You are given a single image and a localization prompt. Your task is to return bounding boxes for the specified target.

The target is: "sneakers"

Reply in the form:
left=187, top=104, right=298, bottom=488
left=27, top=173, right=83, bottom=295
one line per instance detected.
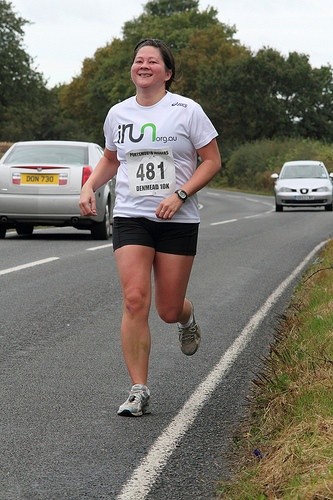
left=176, top=297, right=202, bottom=357
left=117, top=384, right=154, bottom=416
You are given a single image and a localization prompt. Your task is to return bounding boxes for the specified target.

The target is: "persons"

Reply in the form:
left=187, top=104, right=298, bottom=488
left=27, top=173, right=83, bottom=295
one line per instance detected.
left=77, top=38, right=223, bottom=417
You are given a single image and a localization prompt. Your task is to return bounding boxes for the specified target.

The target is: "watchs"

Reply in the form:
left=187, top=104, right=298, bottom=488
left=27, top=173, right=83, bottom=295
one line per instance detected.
left=175, top=189, right=187, bottom=203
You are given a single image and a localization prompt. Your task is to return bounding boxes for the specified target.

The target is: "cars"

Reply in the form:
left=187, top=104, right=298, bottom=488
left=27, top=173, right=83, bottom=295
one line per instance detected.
left=0, top=141, right=116, bottom=241
left=270, top=160, right=333, bottom=212
